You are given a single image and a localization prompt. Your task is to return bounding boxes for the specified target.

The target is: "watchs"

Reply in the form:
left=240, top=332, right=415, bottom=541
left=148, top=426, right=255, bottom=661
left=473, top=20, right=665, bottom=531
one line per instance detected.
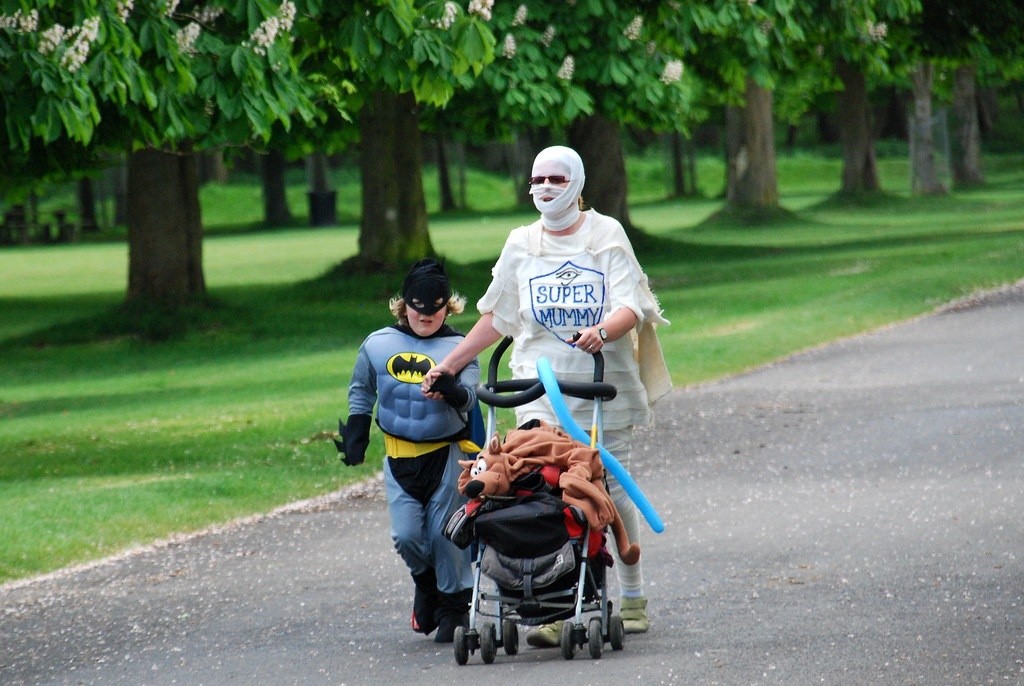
left=597, top=324, right=607, bottom=343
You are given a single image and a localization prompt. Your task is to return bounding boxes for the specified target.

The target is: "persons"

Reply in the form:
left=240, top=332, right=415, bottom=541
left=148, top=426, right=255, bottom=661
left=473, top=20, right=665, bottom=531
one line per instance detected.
left=335, top=263, right=487, bottom=642
left=424, top=146, right=673, bottom=647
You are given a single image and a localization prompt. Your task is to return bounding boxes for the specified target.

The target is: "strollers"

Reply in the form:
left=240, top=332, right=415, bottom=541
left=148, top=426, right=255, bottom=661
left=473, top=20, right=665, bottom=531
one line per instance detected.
left=453, top=332, right=625, bottom=665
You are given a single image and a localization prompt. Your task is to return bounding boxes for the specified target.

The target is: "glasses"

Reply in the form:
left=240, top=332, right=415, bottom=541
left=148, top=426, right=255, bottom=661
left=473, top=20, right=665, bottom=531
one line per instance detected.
left=530, top=175, right=569, bottom=186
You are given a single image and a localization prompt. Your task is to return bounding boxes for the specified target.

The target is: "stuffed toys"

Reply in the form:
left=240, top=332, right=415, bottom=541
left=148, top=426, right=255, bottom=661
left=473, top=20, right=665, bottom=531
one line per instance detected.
left=457, top=426, right=641, bottom=567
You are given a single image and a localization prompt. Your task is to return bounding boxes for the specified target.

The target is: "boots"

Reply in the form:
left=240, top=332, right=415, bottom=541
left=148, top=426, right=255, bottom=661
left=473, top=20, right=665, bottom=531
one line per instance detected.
left=410, top=566, right=474, bottom=643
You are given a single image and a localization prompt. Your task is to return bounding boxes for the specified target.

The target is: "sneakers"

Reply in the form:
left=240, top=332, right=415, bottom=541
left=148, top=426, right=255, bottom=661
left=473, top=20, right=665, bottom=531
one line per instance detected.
left=619, top=595, right=651, bottom=634
left=527, top=620, right=571, bottom=647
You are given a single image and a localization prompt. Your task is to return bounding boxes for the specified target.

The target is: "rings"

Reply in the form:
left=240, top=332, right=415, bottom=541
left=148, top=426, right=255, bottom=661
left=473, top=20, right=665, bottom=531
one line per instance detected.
left=589, top=345, right=594, bottom=351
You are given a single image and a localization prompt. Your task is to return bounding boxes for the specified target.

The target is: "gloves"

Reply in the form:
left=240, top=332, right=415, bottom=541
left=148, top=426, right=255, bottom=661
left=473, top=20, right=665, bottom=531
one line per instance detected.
left=428, top=373, right=469, bottom=408
left=335, top=413, right=370, bottom=467
